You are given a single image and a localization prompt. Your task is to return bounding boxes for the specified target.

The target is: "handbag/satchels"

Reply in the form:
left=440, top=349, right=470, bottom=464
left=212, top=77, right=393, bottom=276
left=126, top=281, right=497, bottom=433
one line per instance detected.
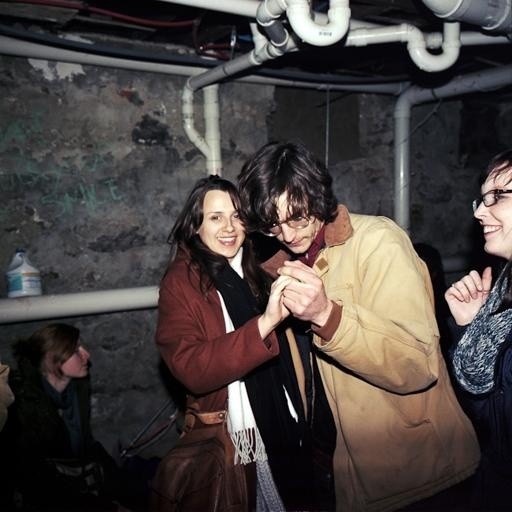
left=114, top=394, right=183, bottom=498
left=150, top=388, right=251, bottom=512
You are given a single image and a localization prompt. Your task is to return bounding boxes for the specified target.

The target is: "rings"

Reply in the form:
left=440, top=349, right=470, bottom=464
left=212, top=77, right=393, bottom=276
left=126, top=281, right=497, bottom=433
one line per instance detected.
left=452, top=281, right=456, bottom=287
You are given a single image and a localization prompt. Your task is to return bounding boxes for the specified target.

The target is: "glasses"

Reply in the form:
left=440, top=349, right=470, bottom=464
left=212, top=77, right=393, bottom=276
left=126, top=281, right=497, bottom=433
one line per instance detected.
left=257, top=206, right=310, bottom=237
left=472, top=188, right=512, bottom=208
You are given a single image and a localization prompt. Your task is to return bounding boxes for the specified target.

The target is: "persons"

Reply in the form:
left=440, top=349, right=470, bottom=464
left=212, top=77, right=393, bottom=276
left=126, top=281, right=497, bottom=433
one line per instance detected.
left=1, top=324, right=130, bottom=512
left=156, top=175, right=302, bottom=512
left=237, top=141, right=487, bottom=512
left=444, top=149, right=512, bottom=512
